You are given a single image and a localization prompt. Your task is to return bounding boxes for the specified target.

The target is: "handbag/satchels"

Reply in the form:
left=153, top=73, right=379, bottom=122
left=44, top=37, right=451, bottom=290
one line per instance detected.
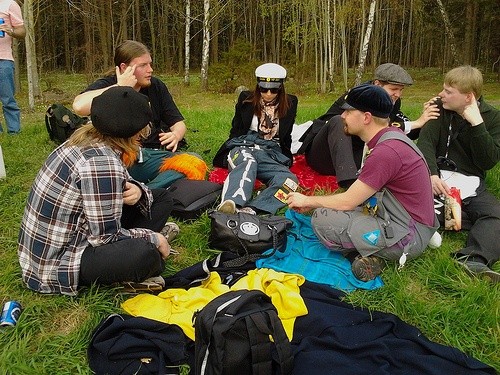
left=162, top=177, right=224, bottom=224
left=207, top=209, right=293, bottom=255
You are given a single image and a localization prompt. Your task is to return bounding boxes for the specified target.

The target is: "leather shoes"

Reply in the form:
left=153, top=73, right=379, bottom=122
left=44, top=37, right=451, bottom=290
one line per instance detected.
left=454, top=259, right=500, bottom=284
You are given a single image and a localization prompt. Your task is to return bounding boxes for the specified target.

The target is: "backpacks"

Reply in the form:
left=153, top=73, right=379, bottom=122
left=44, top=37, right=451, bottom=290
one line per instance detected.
left=192, top=289, right=295, bottom=375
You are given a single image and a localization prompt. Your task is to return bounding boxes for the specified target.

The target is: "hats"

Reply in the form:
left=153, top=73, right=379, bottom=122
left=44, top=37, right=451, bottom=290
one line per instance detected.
left=373, top=63, right=414, bottom=86
left=90, top=85, right=151, bottom=138
left=341, top=84, right=393, bottom=118
left=254, top=63, right=287, bottom=88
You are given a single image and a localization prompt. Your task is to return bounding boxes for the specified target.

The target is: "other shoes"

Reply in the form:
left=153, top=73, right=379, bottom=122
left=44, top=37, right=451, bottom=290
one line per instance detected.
left=351, top=254, right=386, bottom=281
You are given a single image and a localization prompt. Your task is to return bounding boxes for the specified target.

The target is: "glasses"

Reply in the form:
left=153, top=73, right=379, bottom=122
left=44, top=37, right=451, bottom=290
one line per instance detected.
left=259, top=85, right=281, bottom=94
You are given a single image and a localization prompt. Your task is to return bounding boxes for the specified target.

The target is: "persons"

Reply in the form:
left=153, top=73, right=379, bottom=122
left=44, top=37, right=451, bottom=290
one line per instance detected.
left=16, top=84, right=171, bottom=298
left=211, top=62, right=300, bottom=217
left=72, top=40, right=204, bottom=193
left=416, top=63, right=500, bottom=286
left=297, top=61, right=443, bottom=193
left=285, top=83, right=444, bottom=282
left=0, top=1, right=26, bottom=137
